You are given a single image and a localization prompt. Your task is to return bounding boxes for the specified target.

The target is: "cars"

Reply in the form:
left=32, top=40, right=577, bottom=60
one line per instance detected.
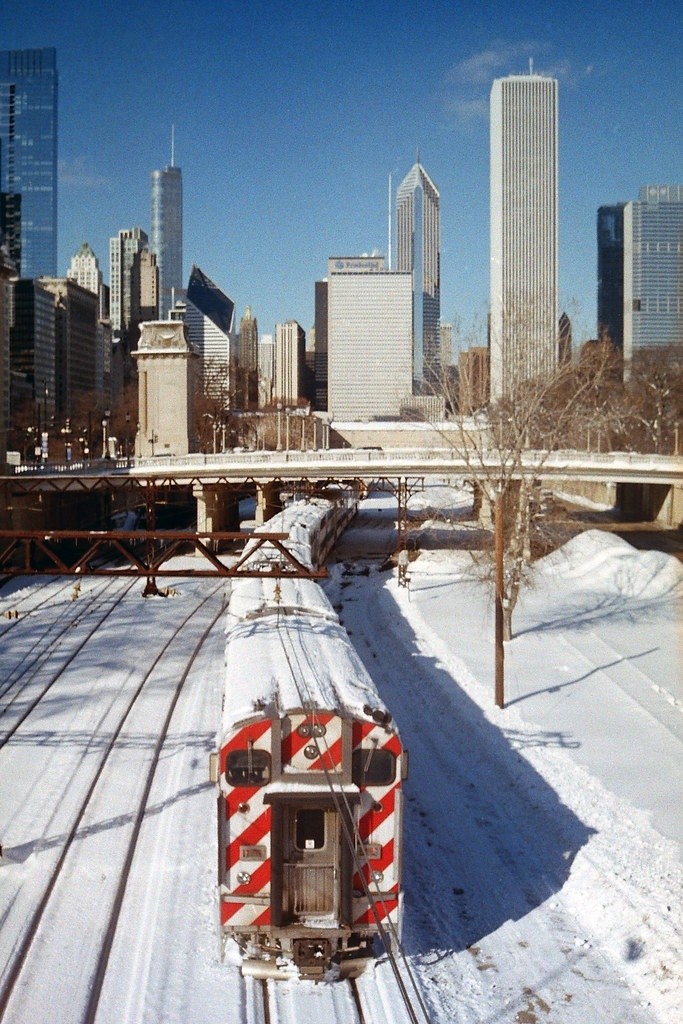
left=354, top=447, right=383, bottom=453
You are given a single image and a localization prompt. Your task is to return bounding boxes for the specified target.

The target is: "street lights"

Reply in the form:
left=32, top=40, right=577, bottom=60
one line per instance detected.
left=313, top=417, right=318, bottom=452
left=322, top=424, right=330, bottom=449
left=221, top=424, right=226, bottom=453
left=285, top=407, right=291, bottom=461
left=301, top=416, right=306, bottom=452
left=203, top=413, right=217, bottom=453
left=276, top=402, right=284, bottom=451
left=101, top=420, right=109, bottom=459
left=124, top=411, right=132, bottom=467
left=212, top=424, right=217, bottom=454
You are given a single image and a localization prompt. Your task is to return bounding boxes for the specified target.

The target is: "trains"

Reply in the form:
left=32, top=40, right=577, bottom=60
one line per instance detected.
left=211, top=477, right=411, bottom=982
